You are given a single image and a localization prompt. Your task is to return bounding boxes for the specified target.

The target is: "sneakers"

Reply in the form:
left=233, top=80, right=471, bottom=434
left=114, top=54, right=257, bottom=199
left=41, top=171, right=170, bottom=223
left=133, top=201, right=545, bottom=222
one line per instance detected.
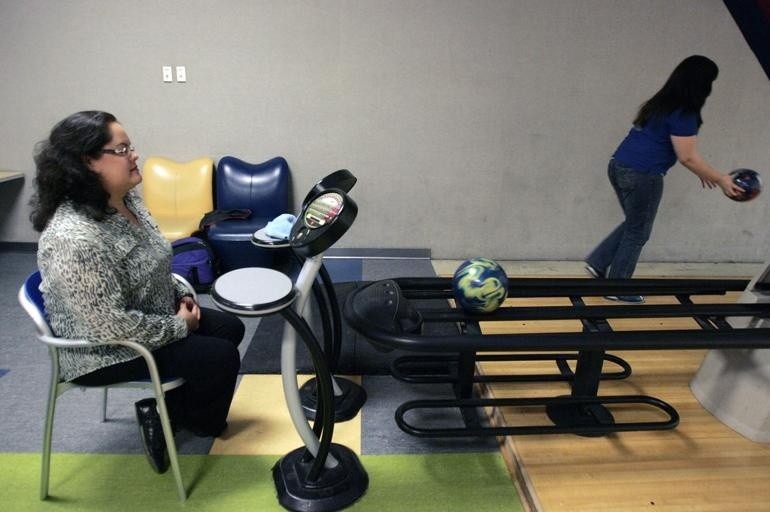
left=163, top=401, right=226, bottom=438
left=583, top=263, right=605, bottom=279
left=605, top=295, right=644, bottom=303
left=135, top=398, right=171, bottom=475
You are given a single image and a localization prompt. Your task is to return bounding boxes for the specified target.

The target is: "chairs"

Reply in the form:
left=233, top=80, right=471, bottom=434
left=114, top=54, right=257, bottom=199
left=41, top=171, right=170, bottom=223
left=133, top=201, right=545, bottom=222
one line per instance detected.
left=17, top=270, right=200, bottom=504
left=141, top=155, right=299, bottom=293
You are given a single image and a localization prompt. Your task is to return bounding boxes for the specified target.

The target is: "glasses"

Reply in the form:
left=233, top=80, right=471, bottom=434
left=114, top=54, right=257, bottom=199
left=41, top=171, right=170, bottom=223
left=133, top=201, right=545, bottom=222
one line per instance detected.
left=97, top=143, right=136, bottom=158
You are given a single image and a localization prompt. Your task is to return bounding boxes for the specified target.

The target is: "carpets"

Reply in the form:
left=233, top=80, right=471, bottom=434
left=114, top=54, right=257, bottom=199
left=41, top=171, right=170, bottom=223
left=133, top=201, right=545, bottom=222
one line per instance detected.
left=0, top=451, right=528, bottom=512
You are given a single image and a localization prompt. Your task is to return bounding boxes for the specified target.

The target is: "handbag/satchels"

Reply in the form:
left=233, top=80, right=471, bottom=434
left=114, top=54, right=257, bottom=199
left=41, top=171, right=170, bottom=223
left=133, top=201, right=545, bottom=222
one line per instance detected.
left=171, top=231, right=220, bottom=295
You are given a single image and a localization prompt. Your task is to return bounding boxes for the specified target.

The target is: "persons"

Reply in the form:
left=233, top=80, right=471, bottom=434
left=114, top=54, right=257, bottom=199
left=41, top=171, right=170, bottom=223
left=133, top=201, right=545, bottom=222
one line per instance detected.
left=585, top=54, right=748, bottom=303
left=29, top=110, right=246, bottom=474
